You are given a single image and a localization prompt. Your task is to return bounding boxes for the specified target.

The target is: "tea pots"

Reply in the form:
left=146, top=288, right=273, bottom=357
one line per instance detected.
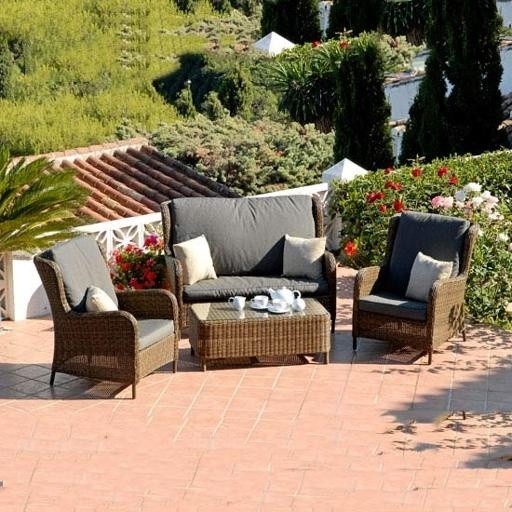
left=270, top=287, right=301, bottom=305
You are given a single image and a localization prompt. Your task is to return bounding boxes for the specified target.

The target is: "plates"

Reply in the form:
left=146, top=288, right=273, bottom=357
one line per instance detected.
left=268, top=308, right=290, bottom=313
left=249, top=304, right=272, bottom=309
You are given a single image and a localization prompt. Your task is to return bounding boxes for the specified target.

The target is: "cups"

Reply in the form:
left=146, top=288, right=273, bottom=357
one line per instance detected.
left=271, top=299, right=288, bottom=310
left=250, top=296, right=268, bottom=306
left=229, top=296, right=245, bottom=309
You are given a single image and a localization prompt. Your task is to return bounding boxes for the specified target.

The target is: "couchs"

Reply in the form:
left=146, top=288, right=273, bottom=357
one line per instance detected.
left=34, top=236, right=180, bottom=399
left=160, top=194, right=338, bottom=334
left=351, top=212, right=479, bottom=364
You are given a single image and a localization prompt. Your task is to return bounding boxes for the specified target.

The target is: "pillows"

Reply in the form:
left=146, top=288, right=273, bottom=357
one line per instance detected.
left=84, top=285, right=119, bottom=315
left=282, top=233, right=326, bottom=282
left=405, top=251, right=455, bottom=302
left=172, top=235, right=216, bottom=285
